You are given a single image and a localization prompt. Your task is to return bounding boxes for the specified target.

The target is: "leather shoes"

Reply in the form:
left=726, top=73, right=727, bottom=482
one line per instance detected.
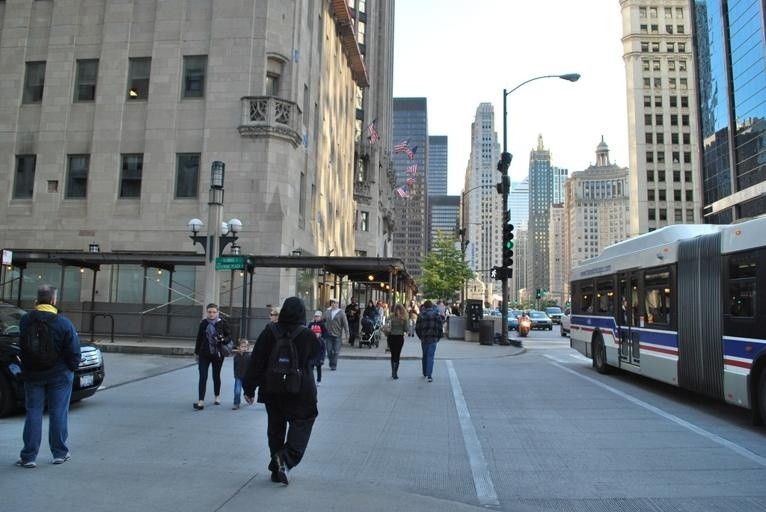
left=215, top=402, right=220, bottom=405
left=193, top=403, right=203, bottom=410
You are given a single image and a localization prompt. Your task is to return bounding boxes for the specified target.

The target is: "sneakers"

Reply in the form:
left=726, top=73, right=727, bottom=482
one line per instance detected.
left=52, top=453, right=71, bottom=464
left=16, top=459, right=36, bottom=468
left=268, top=452, right=289, bottom=484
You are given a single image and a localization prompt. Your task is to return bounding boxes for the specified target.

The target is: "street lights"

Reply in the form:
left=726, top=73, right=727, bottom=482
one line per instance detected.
left=460, top=184, right=497, bottom=312
left=88, top=242, right=100, bottom=340
left=186, top=158, right=243, bottom=344
left=0, top=247, right=12, bottom=301
left=499, top=73, right=581, bottom=340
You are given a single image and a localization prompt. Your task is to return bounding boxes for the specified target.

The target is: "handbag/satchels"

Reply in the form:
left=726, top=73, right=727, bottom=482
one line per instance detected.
left=220, top=339, right=233, bottom=358
left=384, top=320, right=392, bottom=336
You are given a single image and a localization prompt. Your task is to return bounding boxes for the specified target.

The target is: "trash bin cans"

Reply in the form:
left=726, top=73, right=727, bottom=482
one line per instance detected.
left=479, top=320, right=494, bottom=345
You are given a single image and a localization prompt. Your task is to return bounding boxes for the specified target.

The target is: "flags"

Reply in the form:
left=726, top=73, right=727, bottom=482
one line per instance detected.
left=366, top=119, right=417, bottom=200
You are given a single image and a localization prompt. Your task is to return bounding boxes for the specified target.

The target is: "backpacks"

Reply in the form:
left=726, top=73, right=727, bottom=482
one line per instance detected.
left=267, top=322, right=307, bottom=396
left=22, top=311, right=64, bottom=373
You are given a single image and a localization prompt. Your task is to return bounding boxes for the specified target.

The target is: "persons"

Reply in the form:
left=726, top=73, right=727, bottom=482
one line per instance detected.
left=245, top=298, right=323, bottom=486
left=586, top=299, right=671, bottom=328
left=518, top=312, right=532, bottom=320
left=234, top=294, right=460, bottom=410
left=14, top=285, right=80, bottom=469
left=191, top=302, right=231, bottom=410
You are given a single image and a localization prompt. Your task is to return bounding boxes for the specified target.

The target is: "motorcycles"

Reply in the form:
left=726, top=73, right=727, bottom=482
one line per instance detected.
left=516, top=316, right=532, bottom=336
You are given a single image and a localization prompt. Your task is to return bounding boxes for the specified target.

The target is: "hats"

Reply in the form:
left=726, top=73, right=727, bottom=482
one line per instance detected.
left=315, top=311, right=322, bottom=317
left=312, top=325, right=321, bottom=333
left=331, top=299, right=338, bottom=303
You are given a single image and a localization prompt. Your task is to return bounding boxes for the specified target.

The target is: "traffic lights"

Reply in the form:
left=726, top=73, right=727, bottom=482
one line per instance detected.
left=541, top=290, right=546, bottom=296
left=490, top=268, right=500, bottom=278
left=505, top=268, right=512, bottom=279
left=535, top=288, right=541, bottom=299
left=505, top=224, right=514, bottom=266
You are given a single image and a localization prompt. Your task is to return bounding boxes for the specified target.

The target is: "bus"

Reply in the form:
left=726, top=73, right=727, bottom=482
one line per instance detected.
left=569, top=213, right=766, bottom=421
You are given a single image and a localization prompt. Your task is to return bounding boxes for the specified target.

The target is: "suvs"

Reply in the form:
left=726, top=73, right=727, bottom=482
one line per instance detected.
left=560, top=308, right=571, bottom=337
left=0, top=299, right=104, bottom=415
left=545, top=307, right=562, bottom=325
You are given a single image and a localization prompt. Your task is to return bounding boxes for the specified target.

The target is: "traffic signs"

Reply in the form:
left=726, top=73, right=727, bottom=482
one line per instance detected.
left=216, top=257, right=243, bottom=263
left=216, top=264, right=243, bottom=270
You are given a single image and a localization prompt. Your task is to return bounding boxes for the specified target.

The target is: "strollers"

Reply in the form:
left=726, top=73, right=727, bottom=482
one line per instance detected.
left=357, top=318, right=381, bottom=349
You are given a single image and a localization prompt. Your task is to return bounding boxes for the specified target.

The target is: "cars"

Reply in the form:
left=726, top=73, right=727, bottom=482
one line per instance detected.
left=482, top=307, right=553, bottom=331
